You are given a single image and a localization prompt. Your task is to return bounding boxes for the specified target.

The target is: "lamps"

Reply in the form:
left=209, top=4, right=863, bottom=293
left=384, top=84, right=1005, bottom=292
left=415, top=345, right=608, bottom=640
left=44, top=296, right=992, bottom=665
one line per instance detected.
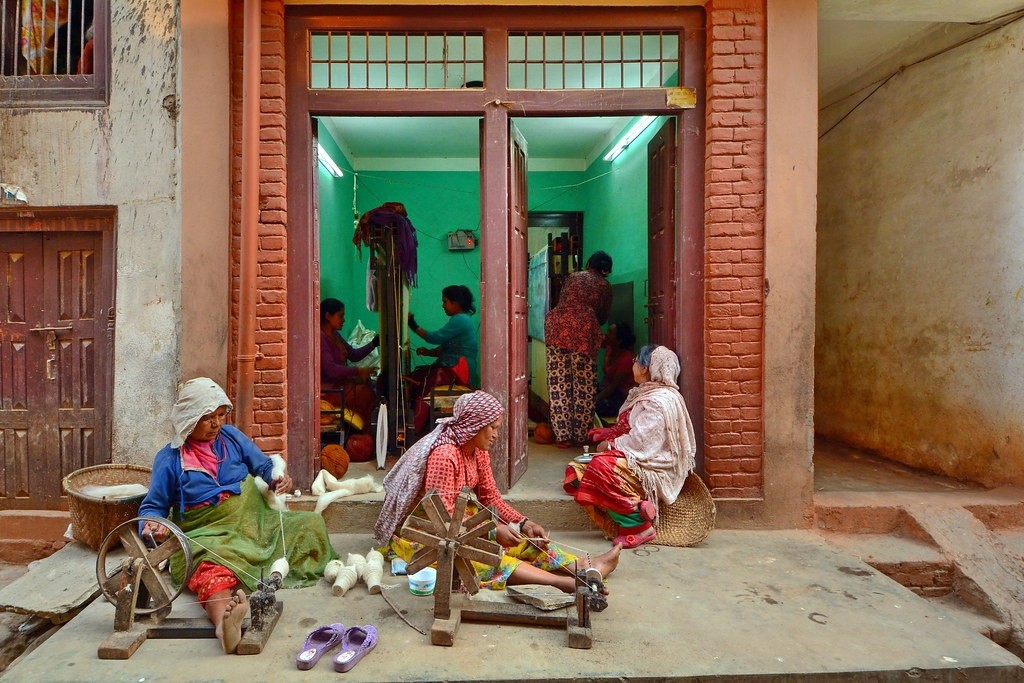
left=318, top=141, right=344, bottom=178
left=603, top=116, right=658, bottom=162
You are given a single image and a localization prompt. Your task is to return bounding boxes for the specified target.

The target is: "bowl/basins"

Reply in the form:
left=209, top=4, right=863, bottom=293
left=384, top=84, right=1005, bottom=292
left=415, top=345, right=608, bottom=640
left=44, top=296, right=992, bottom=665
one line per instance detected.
left=407, top=567, right=438, bottom=596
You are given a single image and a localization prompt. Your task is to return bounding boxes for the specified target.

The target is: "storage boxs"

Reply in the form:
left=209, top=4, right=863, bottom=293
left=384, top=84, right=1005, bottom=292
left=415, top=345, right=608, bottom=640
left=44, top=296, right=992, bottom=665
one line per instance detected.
left=553, top=237, right=563, bottom=252
left=551, top=254, right=561, bottom=273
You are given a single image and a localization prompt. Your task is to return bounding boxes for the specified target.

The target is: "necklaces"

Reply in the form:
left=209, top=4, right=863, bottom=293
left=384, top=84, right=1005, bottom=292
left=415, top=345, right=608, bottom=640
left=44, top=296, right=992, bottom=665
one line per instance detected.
left=184, top=431, right=227, bottom=464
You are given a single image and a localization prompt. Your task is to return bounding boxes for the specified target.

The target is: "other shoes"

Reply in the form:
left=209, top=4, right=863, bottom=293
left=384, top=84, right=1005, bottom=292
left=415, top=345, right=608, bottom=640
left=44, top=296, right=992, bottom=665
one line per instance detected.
left=556, top=439, right=582, bottom=448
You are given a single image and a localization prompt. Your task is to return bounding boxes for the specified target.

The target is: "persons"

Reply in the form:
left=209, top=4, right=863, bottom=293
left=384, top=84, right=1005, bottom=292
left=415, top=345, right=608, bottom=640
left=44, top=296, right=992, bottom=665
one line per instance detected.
left=407, top=285, right=479, bottom=438
left=593, top=320, right=638, bottom=418
left=563, top=343, right=697, bottom=548
left=137, top=377, right=325, bottom=655
left=320, top=297, right=380, bottom=435
left=372, top=389, right=623, bottom=594
left=543, top=250, right=614, bottom=449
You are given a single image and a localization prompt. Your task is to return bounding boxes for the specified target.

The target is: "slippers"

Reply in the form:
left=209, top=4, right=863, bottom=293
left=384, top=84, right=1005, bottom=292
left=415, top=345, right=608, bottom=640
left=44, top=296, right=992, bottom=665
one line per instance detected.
left=297, top=623, right=345, bottom=670
left=639, top=500, right=656, bottom=521
left=613, top=531, right=656, bottom=547
left=333, top=625, right=378, bottom=672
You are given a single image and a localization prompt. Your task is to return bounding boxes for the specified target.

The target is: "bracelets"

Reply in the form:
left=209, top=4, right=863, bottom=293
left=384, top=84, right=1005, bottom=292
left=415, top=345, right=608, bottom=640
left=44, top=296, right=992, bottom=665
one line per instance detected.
left=607, top=441, right=612, bottom=451
left=519, top=518, right=528, bottom=533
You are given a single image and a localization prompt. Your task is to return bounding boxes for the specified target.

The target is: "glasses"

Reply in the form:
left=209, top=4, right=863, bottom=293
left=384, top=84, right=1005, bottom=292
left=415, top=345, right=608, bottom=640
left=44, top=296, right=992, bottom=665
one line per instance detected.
left=633, top=353, right=643, bottom=363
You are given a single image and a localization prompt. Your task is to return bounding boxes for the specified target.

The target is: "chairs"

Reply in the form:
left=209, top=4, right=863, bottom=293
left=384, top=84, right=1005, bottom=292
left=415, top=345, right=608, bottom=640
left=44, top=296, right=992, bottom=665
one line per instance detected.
left=320, top=388, right=348, bottom=451
left=422, top=368, right=477, bottom=434
